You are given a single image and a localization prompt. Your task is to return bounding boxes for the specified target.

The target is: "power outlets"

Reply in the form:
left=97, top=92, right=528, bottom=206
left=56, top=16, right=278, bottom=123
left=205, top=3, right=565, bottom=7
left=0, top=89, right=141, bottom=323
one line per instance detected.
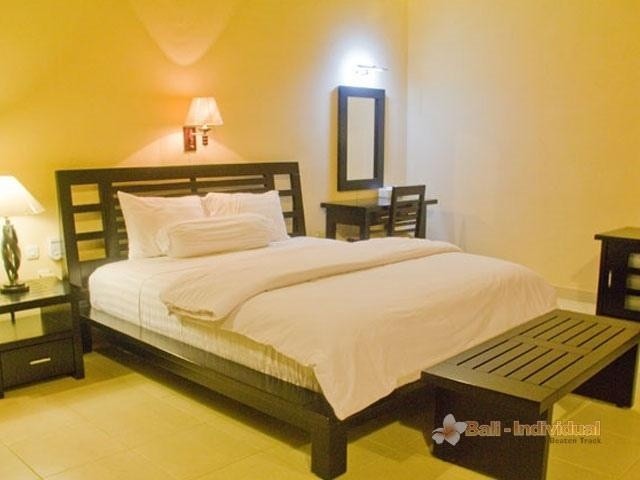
left=25, top=243, right=39, bottom=260
left=48, top=240, right=66, bottom=260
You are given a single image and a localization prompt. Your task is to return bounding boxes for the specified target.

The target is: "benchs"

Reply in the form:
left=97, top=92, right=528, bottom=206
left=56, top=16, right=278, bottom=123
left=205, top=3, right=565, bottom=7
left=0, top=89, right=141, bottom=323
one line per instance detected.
left=421, top=309, right=640, bottom=480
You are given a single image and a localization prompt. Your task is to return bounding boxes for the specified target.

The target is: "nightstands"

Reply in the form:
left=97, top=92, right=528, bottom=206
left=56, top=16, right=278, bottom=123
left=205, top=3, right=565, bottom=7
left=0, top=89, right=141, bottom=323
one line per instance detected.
left=0, top=276, right=85, bottom=399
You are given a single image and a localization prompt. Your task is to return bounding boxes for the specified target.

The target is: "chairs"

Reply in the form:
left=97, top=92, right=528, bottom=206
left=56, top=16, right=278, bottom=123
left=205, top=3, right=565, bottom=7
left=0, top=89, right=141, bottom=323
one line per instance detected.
left=387, top=184, right=426, bottom=239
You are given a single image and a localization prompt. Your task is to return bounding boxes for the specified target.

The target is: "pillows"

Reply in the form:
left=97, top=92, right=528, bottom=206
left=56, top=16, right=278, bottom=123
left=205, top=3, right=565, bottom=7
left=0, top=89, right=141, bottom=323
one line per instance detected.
left=117, top=190, right=205, bottom=260
left=203, top=189, right=291, bottom=244
left=156, top=213, right=276, bottom=260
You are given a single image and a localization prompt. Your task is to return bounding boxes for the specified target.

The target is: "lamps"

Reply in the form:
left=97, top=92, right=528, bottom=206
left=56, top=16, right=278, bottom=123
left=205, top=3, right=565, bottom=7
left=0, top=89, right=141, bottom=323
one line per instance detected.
left=182, top=96, right=223, bottom=152
left=0, top=175, right=46, bottom=295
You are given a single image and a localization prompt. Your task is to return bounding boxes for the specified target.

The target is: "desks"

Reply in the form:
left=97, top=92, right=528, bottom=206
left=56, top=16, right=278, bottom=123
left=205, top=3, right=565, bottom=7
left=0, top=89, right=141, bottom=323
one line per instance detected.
left=320, top=196, right=438, bottom=242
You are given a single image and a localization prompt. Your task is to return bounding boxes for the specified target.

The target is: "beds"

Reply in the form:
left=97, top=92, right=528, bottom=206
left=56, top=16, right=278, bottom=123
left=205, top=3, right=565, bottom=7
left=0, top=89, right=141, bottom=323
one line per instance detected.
left=56, top=161, right=558, bottom=480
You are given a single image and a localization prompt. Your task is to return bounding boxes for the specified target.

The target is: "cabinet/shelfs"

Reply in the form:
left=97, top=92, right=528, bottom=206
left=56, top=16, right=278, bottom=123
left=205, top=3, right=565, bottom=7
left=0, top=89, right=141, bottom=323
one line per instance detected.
left=587, top=227, right=640, bottom=410
